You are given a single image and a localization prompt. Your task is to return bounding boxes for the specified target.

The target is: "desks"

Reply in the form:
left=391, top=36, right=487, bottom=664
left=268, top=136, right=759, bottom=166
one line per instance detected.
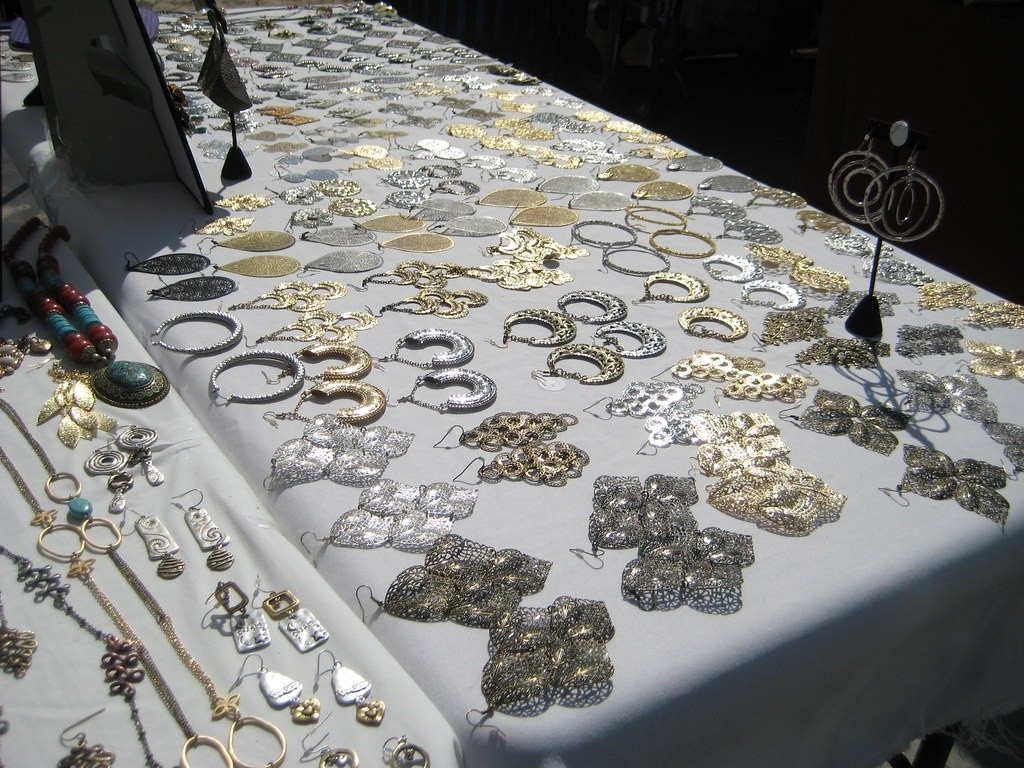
left=0, top=0, right=1024, bottom=768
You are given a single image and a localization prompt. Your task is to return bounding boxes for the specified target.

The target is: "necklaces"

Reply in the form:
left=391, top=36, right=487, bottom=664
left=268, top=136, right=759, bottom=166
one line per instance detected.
left=1, top=218, right=170, bottom=410
left=0, top=396, right=288, bottom=768
